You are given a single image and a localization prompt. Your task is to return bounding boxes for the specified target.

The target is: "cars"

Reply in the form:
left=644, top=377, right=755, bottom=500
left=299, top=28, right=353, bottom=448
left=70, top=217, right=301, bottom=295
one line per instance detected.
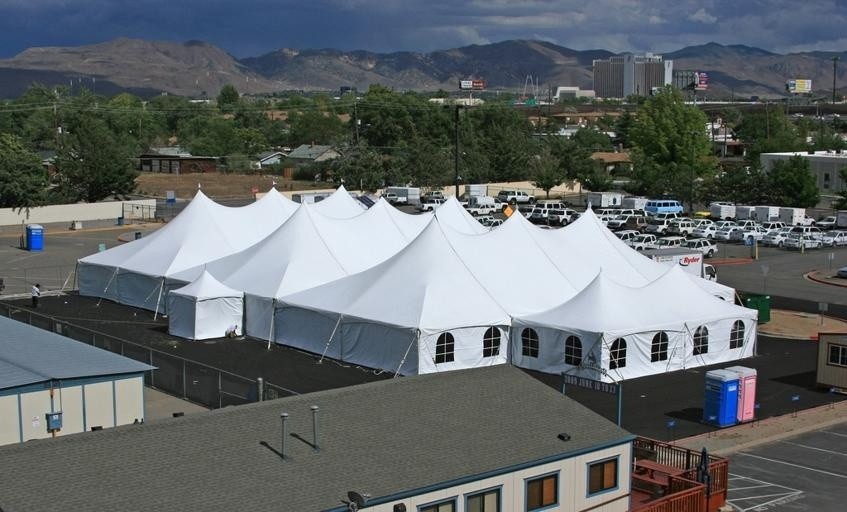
left=593, top=199, right=847, bottom=258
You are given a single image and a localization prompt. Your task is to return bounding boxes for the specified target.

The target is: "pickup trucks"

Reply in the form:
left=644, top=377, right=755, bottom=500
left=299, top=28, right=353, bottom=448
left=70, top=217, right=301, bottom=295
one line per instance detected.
left=380, top=192, right=409, bottom=206
left=414, top=199, right=447, bottom=213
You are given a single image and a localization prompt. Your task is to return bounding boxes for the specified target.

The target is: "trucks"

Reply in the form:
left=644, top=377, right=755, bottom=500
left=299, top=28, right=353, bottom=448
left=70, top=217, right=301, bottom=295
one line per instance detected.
left=636, top=247, right=718, bottom=283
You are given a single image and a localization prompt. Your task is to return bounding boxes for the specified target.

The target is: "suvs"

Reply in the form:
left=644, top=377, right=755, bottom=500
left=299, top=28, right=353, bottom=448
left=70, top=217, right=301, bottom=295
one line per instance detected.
left=421, top=191, right=444, bottom=202
left=462, top=189, right=585, bottom=231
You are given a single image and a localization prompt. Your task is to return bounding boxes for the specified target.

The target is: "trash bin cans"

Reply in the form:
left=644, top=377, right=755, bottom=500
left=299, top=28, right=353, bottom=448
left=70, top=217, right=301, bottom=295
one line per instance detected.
left=135, top=233, right=142, bottom=239
left=25, top=223, right=44, bottom=250
left=118, top=217, right=123, bottom=226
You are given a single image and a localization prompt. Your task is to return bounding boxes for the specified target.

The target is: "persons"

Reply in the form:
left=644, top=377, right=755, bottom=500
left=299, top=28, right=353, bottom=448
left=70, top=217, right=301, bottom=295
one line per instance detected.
left=32, top=283, right=40, bottom=307
